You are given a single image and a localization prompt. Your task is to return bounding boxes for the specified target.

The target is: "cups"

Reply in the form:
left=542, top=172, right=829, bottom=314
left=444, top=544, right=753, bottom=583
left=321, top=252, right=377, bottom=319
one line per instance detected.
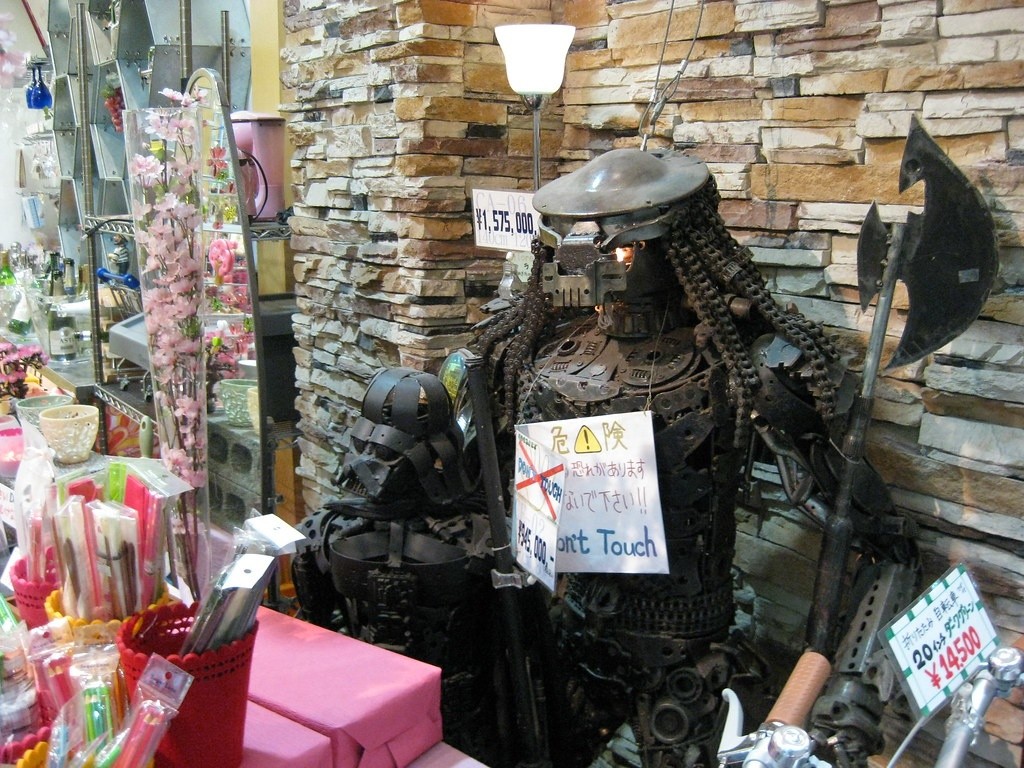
left=45, top=580, right=169, bottom=644
left=10, top=547, right=60, bottom=629
left=117, top=601, right=259, bottom=768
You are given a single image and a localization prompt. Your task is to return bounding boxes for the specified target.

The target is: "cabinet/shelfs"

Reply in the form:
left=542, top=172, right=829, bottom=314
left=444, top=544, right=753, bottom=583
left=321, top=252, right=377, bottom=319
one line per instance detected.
left=84, top=215, right=296, bottom=456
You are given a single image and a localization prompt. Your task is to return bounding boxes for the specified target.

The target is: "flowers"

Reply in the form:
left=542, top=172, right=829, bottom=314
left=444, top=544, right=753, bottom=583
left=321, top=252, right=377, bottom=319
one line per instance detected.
left=0, top=342, right=49, bottom=399
left=122, top=88, right=243, bottom=601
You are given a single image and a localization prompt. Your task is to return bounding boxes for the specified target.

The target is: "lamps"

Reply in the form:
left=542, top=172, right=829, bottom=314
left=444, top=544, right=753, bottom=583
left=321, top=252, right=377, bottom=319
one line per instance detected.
left=494, top=23, right=576, bottom=195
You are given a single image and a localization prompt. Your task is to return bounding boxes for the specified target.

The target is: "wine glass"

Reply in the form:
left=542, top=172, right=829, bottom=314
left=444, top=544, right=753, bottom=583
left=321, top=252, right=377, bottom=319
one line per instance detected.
left=26, top=62, right=52, bottom=109
left=30, top=137, right=59, bottom=180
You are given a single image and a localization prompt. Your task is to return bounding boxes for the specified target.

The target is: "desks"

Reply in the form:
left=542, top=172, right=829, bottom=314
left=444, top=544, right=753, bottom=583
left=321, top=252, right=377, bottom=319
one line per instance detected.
left=0, top=329, right=96, bottom=405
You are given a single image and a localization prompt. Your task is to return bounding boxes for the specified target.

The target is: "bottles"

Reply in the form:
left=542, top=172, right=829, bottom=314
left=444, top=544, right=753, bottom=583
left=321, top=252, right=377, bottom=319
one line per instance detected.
left=0, top=242, right=83, bottom=362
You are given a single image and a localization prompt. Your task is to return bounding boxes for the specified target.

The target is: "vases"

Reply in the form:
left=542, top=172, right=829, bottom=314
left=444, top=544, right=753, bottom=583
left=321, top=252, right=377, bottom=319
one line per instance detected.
left=205, top=381, right=216, bottom=414
